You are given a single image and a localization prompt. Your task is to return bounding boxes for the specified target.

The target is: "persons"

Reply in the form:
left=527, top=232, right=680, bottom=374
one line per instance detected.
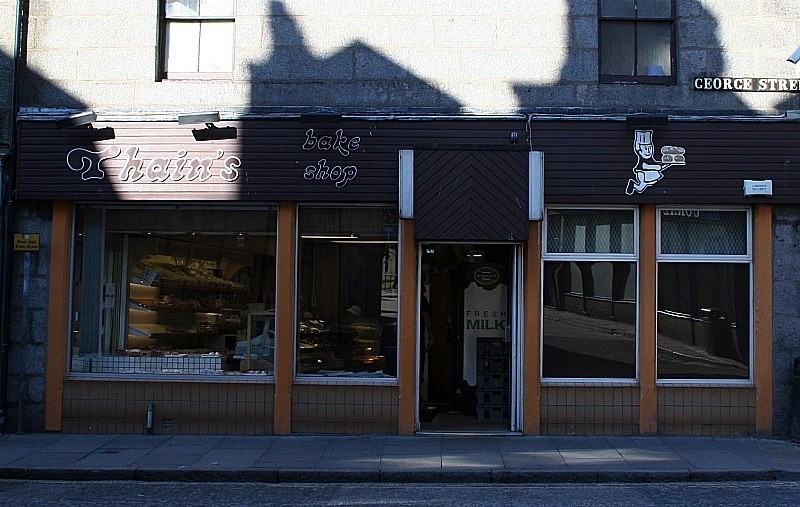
left=420, top=284, right=435, bottom=403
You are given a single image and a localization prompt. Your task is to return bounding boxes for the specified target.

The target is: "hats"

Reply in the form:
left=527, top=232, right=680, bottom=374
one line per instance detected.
left=347, top=305, right=362, bottom=316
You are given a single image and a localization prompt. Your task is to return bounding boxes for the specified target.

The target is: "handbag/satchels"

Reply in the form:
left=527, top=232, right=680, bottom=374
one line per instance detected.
left=423, top=319, right=436, bottom=351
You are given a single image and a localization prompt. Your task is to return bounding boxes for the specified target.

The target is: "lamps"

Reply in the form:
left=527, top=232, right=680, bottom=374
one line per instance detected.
left=54, top=111, right=101, bottom=134
left=179, top=112, right=220, bottom=130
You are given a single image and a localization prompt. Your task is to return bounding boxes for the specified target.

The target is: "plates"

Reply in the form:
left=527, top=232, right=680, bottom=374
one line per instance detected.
left=200, top=354, right=222, bottom=357
left=164, top=354, right=187, bottom=357
